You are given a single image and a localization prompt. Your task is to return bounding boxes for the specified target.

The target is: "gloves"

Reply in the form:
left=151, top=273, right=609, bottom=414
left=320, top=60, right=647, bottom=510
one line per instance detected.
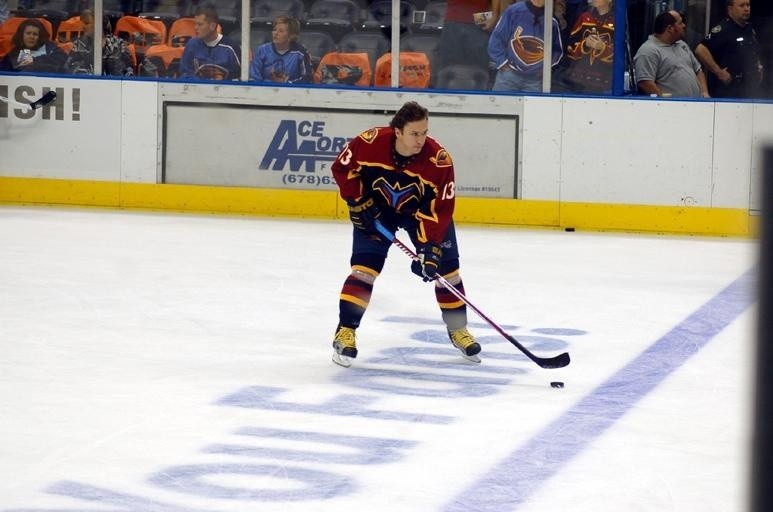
left=347, top=194, right=383, bottom=235
left=411, top=241, right=444, bottom=282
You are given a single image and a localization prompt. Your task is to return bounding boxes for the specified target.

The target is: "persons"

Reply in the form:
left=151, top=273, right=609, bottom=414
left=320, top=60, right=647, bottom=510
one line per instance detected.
left=62, top=7, right=134, bottom=78
left=178, top=5, right=242, bottom=82
left=329, top=100, right=485, bottom=357
left=486, top=0, right=563, bottom=93
left=0, top=19, right=67, bottom=74
left=693, top=0, right=762, bottom=99
left=248, top=16, right=312, bottom=83
left=633, top=11, right=711, bottom=99
left=436, top=1, right=494, bottom=69
left=565, top=0, right=615, bottom=94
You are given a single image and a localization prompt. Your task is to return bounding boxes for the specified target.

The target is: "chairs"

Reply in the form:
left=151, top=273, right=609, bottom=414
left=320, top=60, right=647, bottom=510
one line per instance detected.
left=0, top=0, right=497, bottom=93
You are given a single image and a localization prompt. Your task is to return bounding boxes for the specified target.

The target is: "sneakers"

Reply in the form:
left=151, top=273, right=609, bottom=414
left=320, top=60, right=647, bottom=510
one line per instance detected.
left=333, top=324, right=358, bottom=359
left=447, top=326, right=481, bottom=357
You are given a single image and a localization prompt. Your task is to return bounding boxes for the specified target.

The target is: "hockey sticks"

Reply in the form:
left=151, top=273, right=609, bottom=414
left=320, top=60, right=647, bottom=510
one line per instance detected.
left=375, top=220, right=570, bottom=368
